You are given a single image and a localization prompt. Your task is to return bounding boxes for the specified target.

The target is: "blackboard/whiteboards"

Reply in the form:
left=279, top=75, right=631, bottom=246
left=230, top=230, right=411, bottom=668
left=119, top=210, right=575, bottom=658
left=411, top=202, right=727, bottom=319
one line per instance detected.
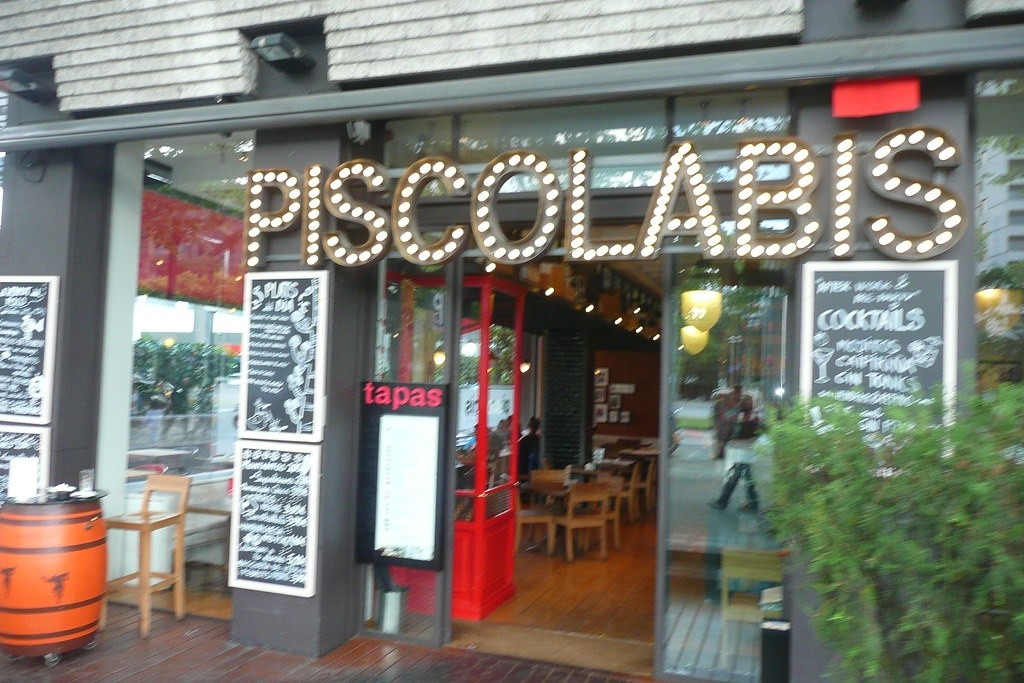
left=795, top=260, right=962, bottom=491
left=234, top=271, right=327, bottom=442
left=225, top=442, right=326, bottom=598
left=0, top=275, right=63, bottom=507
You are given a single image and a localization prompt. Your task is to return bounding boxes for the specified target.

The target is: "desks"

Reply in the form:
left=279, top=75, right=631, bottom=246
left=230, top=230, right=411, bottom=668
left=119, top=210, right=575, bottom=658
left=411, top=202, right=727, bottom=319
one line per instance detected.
left=530, top=469, right=624, bottom=541
left=595, top=458, right=636, bottom=507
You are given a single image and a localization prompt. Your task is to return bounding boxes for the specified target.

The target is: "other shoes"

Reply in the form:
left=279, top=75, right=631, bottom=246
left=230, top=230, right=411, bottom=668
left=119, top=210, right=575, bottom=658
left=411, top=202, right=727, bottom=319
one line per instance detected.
left=709, top=502, right=727, bottom=510
left=738, top=501, right=758, bottom=513
left=713, top=454, right=724, bottom=459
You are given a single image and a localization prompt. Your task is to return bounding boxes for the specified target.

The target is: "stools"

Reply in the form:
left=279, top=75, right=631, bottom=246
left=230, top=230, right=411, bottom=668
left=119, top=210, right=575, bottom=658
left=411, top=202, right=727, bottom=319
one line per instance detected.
left=97, top=474, right=193, bottom=638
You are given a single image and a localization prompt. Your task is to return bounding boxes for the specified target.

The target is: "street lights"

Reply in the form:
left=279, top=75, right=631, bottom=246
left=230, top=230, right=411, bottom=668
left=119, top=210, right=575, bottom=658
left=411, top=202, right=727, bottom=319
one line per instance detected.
left=726, top=333, right=742, bottom=388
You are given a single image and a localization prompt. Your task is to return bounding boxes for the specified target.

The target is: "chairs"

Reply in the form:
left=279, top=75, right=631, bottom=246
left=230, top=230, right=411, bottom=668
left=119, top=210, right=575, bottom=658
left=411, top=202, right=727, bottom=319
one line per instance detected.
left=577, top=476, right=625, bottom=552
left=621, top=461, right=642, bottom=522
left=557, top=482, right=611, bottom=562
left=625, top=458, right=657, bottom=508
left=720, top=547, right=787, bottom=651
left=515, top=481, right=555, bottom=557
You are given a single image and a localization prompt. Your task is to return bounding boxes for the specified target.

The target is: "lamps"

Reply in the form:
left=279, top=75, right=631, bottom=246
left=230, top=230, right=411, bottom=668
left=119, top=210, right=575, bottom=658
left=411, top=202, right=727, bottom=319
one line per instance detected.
left=0, top=70, right=47, bottom=99
left=249, top=32, right=301, bottom=62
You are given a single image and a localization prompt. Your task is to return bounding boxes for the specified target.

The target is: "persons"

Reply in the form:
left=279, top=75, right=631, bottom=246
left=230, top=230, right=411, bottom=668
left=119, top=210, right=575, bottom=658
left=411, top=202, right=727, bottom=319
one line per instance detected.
left=455, top=414, right=513, bottom=519
left=518, top=417, right=540, bottom=504
left=710, top=385, right=759, bottom=512
left=161, top=378, right=193, bottom=442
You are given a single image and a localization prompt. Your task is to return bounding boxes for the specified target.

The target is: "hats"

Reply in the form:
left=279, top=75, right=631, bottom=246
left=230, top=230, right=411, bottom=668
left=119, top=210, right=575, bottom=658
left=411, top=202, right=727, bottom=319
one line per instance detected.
left=744, top=388, right=762, bottom=411
